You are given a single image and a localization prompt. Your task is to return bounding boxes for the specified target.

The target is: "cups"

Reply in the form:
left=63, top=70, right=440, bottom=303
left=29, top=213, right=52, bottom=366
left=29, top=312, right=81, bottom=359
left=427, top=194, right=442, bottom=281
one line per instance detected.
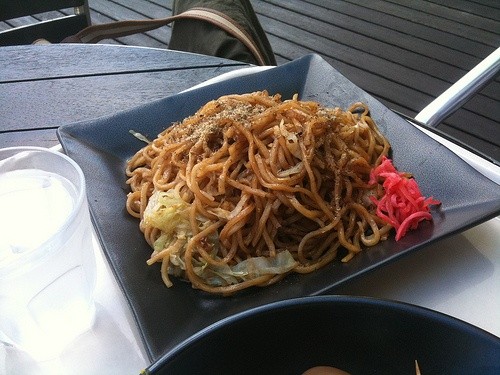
left=1, top=146, right=96, bottom=350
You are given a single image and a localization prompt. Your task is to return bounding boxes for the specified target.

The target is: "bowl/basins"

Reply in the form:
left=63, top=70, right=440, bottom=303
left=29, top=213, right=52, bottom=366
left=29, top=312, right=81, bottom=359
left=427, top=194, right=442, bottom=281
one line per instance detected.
left=143, top=295, right=499, bottom=375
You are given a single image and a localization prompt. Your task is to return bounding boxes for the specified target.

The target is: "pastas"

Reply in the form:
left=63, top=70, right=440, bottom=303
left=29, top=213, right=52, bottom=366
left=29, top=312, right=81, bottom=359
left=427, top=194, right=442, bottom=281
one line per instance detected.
left=125, top=90, right=439, bottom=293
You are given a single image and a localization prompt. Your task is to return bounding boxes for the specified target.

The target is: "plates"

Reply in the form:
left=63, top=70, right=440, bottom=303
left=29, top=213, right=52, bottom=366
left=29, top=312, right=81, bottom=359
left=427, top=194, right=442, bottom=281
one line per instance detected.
left=55, top=53, right=500, bottom=363
